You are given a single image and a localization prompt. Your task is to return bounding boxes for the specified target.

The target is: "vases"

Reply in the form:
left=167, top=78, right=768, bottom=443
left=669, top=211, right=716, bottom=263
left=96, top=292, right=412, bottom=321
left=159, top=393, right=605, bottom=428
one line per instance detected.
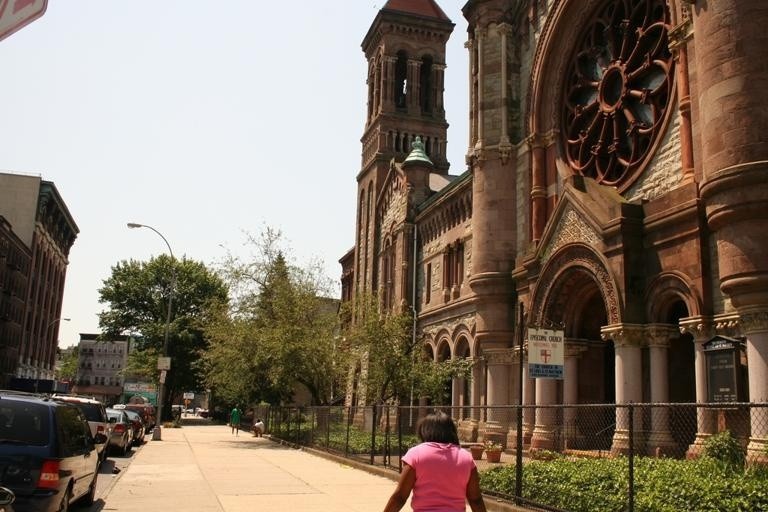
left=470, top=445, right=485, bottom=461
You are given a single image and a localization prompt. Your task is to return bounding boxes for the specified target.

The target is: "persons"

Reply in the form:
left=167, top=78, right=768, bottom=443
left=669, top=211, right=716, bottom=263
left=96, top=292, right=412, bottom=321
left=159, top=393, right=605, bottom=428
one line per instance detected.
left=230, top=403, right=242, bottom=436
left=382, top=409, right=487, bottom=511
left=252, top=418, right=265, bottom=437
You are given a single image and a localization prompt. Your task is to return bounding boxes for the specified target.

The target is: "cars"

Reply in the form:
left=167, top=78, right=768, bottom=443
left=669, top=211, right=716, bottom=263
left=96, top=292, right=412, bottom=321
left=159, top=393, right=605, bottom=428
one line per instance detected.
left=52, top=395, right=116, bottom=469
left=1, top=396, right=108, bottom=512
left=104, top=402, right=180, bottom=454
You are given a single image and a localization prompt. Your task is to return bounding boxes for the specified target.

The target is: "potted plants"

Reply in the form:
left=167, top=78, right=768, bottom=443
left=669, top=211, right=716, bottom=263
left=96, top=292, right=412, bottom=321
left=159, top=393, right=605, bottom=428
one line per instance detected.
left=485, top=440, right=502, bottom=463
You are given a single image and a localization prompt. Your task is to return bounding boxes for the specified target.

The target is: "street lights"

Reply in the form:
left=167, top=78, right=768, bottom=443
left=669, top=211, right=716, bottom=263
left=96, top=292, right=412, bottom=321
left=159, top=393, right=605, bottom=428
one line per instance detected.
left=35, top=319, right=70, bottom=393
left=128, top=224, right=173, bottom=426
